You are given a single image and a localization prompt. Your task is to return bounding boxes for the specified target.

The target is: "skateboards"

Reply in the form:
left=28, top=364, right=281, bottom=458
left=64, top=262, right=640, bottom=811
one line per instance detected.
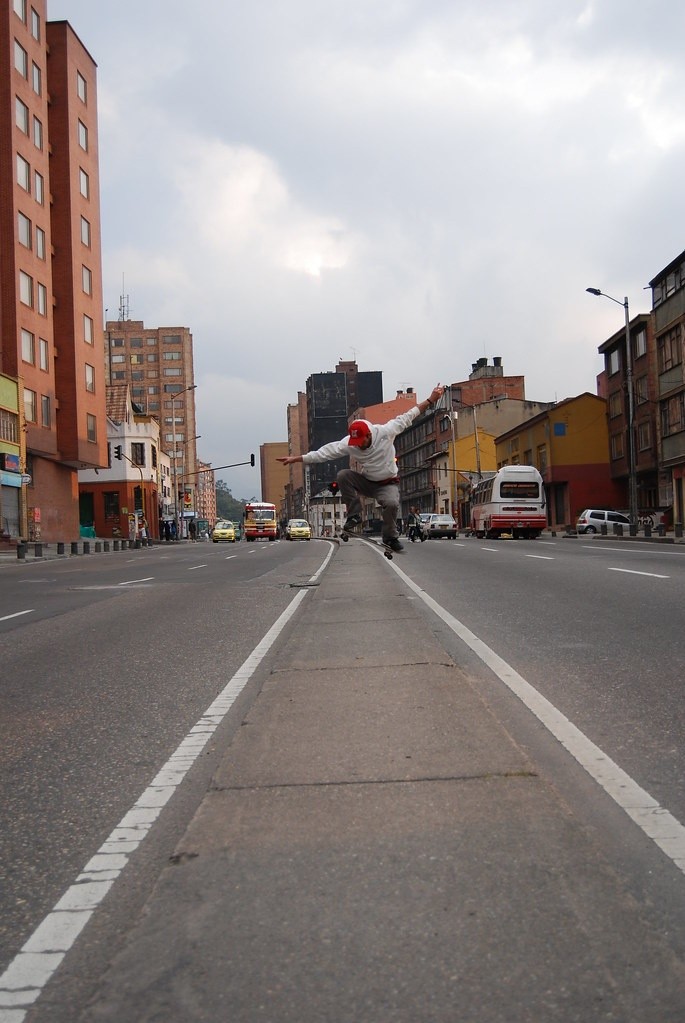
left=331, top=520, right=407, bottom=560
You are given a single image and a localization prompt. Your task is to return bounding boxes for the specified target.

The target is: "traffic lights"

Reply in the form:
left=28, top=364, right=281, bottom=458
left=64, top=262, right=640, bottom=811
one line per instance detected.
left=113, top=445, right=122, bottom=460
left=328, top=482, right=339, bottom=494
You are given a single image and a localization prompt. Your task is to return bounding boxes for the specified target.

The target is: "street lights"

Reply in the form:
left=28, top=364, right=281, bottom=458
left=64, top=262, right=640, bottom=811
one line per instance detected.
left=444, top=414, right=460, bottom=527
left=586, top=287, right=639, bottom=529
left=450, top=398, right=481, bottom=480
left=171, top=385, right=198, bottom=539
left=181, top=435, right=202, bottom=540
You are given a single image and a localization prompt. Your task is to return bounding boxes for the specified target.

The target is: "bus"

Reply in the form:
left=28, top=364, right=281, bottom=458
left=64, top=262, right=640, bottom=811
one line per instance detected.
left=468, top=465, right=548, bottom=539
left=242, top=503, right=278, bottom=542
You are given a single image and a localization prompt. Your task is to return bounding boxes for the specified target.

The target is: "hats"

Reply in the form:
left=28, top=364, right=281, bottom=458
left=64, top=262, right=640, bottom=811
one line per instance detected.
left=348, top=421, right=369, bottom=446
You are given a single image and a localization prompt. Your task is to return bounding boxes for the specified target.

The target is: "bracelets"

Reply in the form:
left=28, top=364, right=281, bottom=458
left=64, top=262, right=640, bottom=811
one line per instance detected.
left=427, top=398, right=433, bottom=406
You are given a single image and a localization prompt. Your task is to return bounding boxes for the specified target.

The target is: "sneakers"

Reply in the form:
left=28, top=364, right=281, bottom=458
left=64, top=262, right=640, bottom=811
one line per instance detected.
left=383, top=538, right=403, bottom=550
left=344, top=514, right=362, bottom=529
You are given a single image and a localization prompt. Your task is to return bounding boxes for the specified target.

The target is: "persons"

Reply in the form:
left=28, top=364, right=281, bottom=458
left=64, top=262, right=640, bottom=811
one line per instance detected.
left=138, top=524, right=146, bottom=538
left=159, top=520, right=178, bottom=541
left=276, top=382, right=446, bottom=551
left=277, top=525, right=291, bottom=540
left=406, top=506, right=426, bottom=543
left=189, top=518, right=196, bottom=540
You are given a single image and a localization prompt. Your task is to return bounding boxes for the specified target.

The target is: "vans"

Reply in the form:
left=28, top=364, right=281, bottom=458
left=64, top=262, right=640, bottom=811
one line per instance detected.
left=285, top=518, right=305, bottom=540
left=576, top=509, right=630, bottom=534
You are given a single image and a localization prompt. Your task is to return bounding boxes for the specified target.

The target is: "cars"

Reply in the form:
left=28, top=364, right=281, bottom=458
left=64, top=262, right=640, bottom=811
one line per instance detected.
left=421, top=514, right=458, bottom=539
left=416, top=513, right=437, bottom=534
left=231, top=521, right=242, bottom=540
left=212, top=521, right=236, bottom=543
left=289, top=520, right=311, bottom=541
